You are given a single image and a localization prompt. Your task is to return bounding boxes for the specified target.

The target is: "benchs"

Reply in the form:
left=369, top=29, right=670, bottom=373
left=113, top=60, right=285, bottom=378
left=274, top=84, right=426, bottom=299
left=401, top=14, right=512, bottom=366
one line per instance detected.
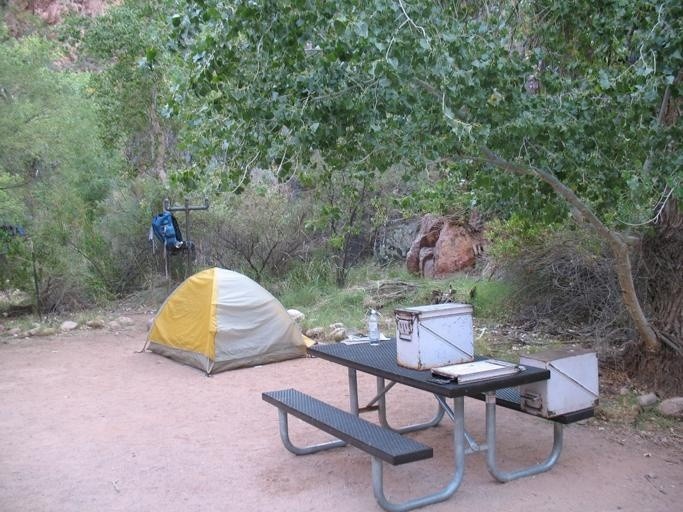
left=476, top=388, right=595, bottom=458
left=262, top=388, right=434, bottom=512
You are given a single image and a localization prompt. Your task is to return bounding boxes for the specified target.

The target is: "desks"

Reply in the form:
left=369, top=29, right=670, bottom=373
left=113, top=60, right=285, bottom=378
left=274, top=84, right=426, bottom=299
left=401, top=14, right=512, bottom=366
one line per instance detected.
left=306, top=337, right=551, bottom=476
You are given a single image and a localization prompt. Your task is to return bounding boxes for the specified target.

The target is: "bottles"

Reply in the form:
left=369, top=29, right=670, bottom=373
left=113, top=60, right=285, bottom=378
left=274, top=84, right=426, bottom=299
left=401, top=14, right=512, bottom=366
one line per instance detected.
left=368, top=310, right=380, bottom=345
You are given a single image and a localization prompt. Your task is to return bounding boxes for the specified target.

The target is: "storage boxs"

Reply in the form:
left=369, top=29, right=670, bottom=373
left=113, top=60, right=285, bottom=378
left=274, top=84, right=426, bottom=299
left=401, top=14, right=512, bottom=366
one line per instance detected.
left=395, top=302, right=474, bottom=370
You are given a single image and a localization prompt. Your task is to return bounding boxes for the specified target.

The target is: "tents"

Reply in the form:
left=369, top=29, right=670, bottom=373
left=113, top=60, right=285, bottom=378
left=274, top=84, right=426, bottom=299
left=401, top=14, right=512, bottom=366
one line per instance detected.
left=141, top=268, right=307, bottom=377
